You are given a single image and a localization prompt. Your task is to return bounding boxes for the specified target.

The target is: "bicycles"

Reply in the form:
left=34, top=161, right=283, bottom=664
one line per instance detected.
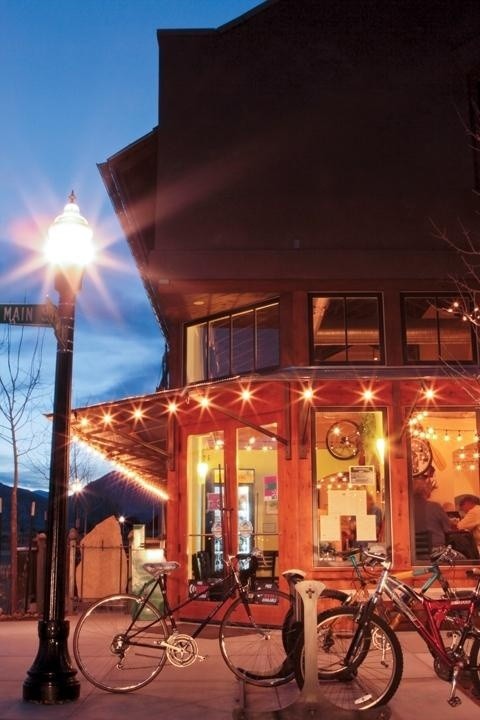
left=73, top=550, right=305, bottom=693
left=280, top=546, right=478, bottom=716
left=326, top=411, right=434, bottom=479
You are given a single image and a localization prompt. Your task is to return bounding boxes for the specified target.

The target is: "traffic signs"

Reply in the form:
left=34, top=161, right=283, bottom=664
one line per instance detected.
left=0, top=302, right=54, bottom=325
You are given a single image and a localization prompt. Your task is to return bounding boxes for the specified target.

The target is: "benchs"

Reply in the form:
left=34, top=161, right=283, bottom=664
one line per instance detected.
left=345, top=587, right=474, bottom=622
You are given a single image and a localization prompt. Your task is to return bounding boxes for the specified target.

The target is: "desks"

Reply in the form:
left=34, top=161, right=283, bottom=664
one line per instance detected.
left=445, top=531, right=480, bottom=559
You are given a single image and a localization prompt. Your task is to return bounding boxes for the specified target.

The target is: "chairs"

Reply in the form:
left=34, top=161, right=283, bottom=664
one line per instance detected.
left=197, top=551, right=224, bottom=595
left=250, top=551, right=275, bottom=603
left=415, top=530, right=431, bottom=560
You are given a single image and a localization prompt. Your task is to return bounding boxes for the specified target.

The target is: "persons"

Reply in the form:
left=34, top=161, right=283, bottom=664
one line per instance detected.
left=457, top=497, right=480, bottom=557
left=412, top=479, right=453, bottom=560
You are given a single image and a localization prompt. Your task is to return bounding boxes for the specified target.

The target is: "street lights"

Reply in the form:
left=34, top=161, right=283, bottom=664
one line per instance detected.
left=21, top=191, right=93, bottom=703
